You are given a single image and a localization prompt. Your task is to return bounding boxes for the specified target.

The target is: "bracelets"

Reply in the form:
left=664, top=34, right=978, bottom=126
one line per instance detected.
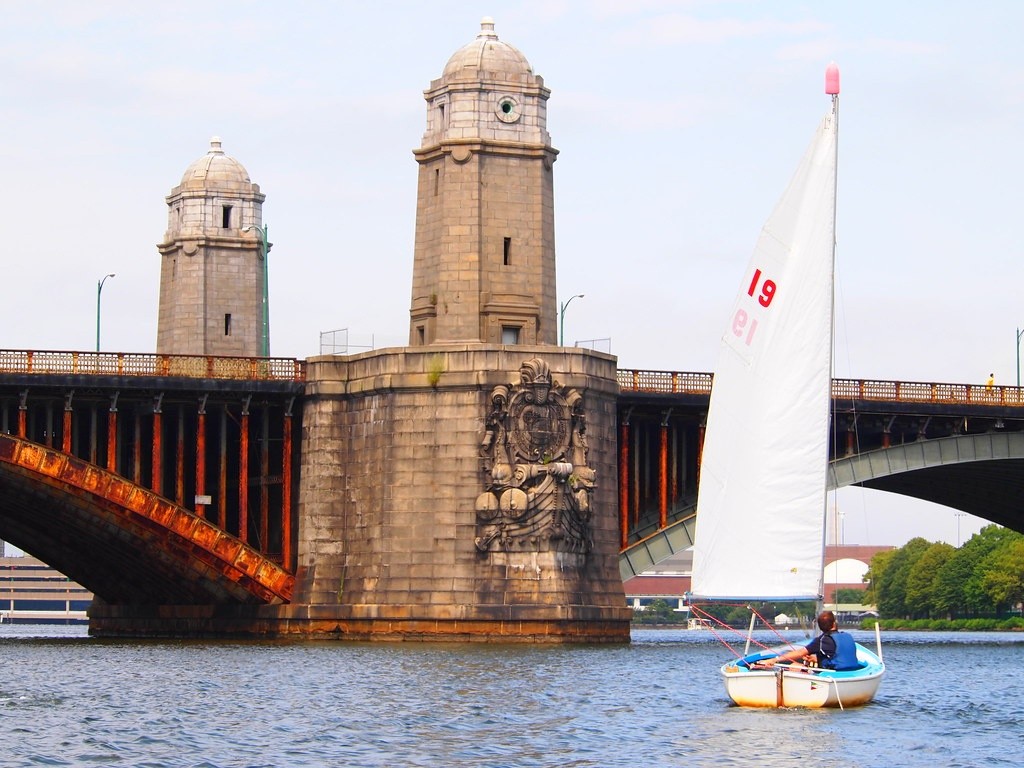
left=775, top=656, right=780, bottom=663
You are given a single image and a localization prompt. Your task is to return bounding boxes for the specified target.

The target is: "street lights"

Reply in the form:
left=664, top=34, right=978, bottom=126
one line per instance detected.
left=96, top=273, right=117, bottom=352
left=559, top=294, right=584, bottom=347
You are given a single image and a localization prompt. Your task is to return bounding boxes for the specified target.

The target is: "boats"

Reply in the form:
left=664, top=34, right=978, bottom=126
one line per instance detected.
left=684, top=616, right=715, bottom=631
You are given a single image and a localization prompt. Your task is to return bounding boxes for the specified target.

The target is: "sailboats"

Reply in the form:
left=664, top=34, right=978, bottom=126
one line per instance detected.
left=685, top=58, right=887, bottom=709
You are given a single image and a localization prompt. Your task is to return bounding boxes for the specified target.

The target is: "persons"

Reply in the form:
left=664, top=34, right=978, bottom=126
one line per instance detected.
left=982, top=374, right=997, bottom=402
left=758, top=611, right=859, bottom=672
